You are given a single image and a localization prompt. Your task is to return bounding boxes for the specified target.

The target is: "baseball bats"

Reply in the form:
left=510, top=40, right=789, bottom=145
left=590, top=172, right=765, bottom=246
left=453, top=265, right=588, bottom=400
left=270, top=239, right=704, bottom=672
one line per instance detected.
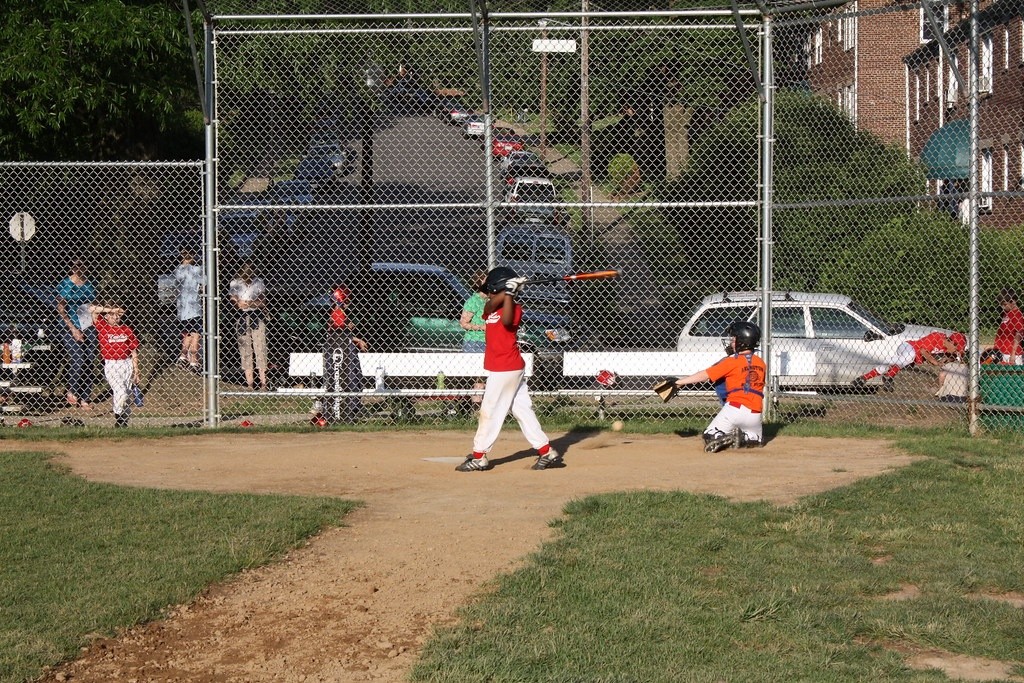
left=517, top=269, right=624, bottom=286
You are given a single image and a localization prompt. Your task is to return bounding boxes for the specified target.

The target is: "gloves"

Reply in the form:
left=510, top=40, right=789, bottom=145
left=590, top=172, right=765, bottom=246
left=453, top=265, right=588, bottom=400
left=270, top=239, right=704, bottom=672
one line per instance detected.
left=504, top=277, right=527, bottom=296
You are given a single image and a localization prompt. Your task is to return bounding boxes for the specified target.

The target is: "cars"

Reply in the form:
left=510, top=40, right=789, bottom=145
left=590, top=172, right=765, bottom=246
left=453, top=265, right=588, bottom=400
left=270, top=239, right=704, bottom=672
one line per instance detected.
left=224, top=90, right=442, bottom=256
left=448, top=105, right=572, bottom=275
left=298, top=264, right=576, bottom=357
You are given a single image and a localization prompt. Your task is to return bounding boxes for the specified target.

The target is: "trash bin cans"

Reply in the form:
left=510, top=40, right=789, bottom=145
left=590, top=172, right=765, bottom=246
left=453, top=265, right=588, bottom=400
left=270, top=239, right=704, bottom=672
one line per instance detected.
left=979, top=363, right=1023, bottom=433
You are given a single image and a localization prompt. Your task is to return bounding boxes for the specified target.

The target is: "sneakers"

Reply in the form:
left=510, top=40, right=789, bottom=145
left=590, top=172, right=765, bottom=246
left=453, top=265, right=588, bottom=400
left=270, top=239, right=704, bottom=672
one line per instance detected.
left=530, top=447, right=563, bottom=469
left=702, top=426, right=734, bottom=453
left=882, top=373, right=894, bottom=393
left=733, top=426, right=760, bottom=449
left=454, top=453, right=489, bottom=472
left=850, top=376, right=866, bottom=388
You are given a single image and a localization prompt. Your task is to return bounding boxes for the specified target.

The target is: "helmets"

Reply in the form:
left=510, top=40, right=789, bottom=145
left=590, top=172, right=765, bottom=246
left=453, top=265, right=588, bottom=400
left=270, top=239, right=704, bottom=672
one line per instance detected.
left=478, top=266, right=518, bottom=294
left=721, top=321, right=760, bottom=357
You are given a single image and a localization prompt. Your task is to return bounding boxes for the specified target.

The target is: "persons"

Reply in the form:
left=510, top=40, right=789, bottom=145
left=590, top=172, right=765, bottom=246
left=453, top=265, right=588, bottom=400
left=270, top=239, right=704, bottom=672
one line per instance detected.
left=230, top=264, right=267, bottom=392
left=936, top=348, right=1017, bottom=403
left=460, top=273, right=491, bottom=422
left=91, top=300, right=140, bottom=428
left=174, top=250, right=202, bottom=366
left=994, top=287, right=1024, bottom=365
left=57, top=258, right=96, bottom=411
left=324, top=285, right=368, bottom=423
left=654, top=322, right=766, bottom=453
left=455, top=266, right=561, bottom=472
left=850, top=332, right=967, bottom=392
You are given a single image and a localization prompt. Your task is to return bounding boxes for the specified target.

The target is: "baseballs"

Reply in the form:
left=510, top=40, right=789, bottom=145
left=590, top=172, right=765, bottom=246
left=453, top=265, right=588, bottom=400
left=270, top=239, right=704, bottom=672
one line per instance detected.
left=612, top=420, right=626, bottom=431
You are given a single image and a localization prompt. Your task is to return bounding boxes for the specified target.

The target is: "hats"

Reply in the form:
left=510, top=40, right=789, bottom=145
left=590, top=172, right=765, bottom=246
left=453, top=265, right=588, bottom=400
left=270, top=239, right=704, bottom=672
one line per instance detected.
left=949, top=332, right=967, bottom=355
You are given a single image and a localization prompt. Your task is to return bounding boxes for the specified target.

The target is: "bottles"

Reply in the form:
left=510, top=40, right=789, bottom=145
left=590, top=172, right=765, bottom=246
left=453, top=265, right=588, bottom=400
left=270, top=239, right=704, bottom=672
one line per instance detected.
left=133, top=383, right=142, bottom=407
left=376, top=367, right=383, bottom=392
left=437, top=371, right=444, bottom=389
left=3, top=343, right=10, bottom=364
left=310, top=372, right=315, bottom=389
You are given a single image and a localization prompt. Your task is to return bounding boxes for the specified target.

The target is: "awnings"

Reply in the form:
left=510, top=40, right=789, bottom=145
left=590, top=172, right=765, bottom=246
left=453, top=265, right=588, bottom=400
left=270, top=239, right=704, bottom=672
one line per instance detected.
left=920, top=117, right=969, bottom=179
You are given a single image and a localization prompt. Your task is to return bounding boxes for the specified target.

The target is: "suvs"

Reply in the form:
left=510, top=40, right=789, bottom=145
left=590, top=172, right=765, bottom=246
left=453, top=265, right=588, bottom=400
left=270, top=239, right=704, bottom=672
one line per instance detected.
left=676, top=290, right=970, bottom=383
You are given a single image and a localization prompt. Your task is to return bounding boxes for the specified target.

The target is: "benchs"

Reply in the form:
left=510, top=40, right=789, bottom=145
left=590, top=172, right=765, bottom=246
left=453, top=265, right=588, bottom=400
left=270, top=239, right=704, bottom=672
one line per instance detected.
left=274, top=351, right=821, bottom=420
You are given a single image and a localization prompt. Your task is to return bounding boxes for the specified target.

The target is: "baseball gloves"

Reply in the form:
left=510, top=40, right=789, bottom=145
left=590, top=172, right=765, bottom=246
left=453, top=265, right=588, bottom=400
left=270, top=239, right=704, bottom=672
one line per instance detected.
left=654, top=377, right=685, bottom=403
